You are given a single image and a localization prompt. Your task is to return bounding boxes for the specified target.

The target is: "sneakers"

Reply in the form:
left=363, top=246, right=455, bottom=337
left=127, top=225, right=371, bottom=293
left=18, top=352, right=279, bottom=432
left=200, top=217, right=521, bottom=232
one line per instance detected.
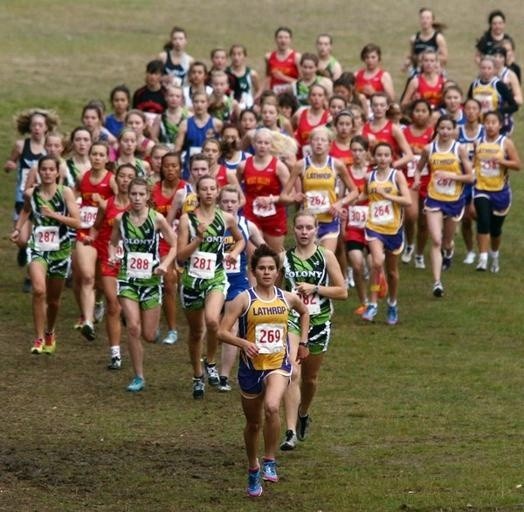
left=280, top=429, right=298, bottom=451
left=262, top=455, right=279, bottom=483
left=218, top=375, right=232, bottom=391
left=343, top=244, right=501, bottom=326
left=30, top=301, right=179, bottom=370
left=126, top=376, right=146, bottom=392
left=297, top=403, right=309, bottom=440
left=192, top=375, right=206, bottom=400
left=203, top=357, right=220, bottom=387
left=247, top=459, right=263, bottom=498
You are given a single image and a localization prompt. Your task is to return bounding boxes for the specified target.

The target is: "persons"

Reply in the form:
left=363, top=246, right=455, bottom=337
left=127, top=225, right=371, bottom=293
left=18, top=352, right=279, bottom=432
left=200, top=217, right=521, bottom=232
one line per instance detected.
left=4, top=26, right=523, bottom=394
left=401, top=7, right=450, bottom=81
left=274, top=208, right=349, bottom=450
left=176, top=174, right=246, bottom=399
left=216, top=244, right=311, bottom=496
left=474, top=9, right=509, bottom=64
